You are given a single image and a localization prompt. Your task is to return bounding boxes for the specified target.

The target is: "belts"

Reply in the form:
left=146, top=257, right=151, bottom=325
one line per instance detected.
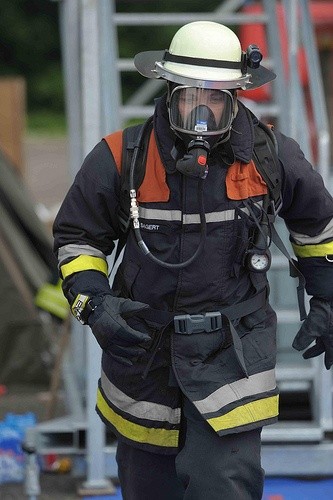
left=134, top=287, right=267, bottom=378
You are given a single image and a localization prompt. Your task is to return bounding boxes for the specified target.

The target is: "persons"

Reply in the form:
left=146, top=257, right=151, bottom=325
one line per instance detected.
left=51, top=19, right=333, bottom=500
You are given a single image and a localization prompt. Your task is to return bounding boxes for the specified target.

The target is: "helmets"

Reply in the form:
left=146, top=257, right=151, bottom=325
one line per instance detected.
left=133, top=20, right=277, bottom=90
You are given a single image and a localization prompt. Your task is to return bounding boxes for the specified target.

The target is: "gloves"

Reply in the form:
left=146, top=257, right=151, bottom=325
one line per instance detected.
left=292, top=293, right=333, bottom=370
left=80, top=294, right=151, bottom=369
